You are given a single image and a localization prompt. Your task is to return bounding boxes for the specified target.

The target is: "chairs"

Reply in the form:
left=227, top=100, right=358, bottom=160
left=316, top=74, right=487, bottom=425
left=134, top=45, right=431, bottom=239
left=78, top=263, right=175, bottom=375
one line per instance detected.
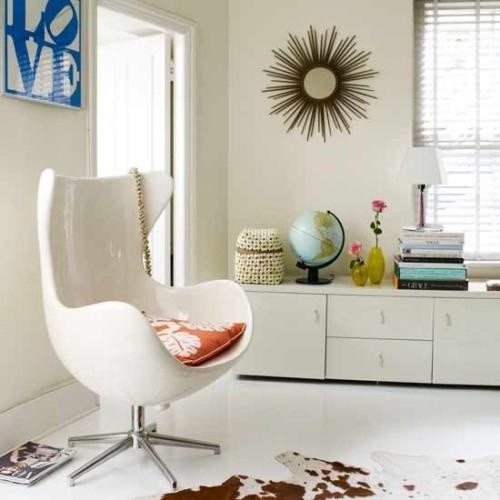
left=40, top=166, right=254, bottom=486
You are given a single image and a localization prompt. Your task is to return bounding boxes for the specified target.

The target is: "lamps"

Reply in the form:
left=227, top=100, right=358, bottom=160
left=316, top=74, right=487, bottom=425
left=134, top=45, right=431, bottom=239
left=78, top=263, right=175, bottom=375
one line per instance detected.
left=396, top=146, right=446, bottom=232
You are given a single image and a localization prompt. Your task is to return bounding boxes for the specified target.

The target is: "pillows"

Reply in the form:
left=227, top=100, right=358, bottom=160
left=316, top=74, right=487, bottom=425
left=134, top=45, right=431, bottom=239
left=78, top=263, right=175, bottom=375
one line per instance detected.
left=142, top=309, right=246, bottom=365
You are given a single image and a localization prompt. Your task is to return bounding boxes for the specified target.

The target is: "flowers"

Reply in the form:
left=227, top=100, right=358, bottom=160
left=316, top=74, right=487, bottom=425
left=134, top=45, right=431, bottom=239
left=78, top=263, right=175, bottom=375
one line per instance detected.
left=349, top=240, right=364, bottom=268
left=372, top=200, right=387, bottom=247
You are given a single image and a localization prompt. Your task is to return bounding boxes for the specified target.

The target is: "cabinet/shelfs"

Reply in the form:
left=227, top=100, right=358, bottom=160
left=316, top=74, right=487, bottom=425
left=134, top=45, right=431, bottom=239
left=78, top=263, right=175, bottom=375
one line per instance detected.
left=235, top=292, right=327, bottom=381
left=327, top=296, right=433, bottom=386
left=433, top=298, right=500, bottom=388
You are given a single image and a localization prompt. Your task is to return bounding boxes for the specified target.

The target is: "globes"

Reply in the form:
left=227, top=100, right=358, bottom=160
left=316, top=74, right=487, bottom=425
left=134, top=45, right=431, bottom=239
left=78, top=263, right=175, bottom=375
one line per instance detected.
left=288, top=209, right=345, bottom=284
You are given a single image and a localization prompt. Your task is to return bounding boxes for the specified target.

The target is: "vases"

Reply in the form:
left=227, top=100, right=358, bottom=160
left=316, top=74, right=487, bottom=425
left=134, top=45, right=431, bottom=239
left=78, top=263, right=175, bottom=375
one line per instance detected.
left=351, top=260, right=368, bottom=286
left=366, top=247, right=385, bottom=286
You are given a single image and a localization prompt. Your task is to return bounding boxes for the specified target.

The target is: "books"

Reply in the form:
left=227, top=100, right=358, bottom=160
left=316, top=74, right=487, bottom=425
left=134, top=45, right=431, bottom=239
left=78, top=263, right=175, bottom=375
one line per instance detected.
left=392, top=228, right=469, bottom=292
left=0, top=440, right=77, bottom=487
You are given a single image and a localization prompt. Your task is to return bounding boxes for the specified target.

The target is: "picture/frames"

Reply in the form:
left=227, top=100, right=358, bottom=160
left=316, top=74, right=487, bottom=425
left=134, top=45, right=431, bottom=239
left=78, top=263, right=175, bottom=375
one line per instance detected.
left=1, top=0, right=84, bottom=112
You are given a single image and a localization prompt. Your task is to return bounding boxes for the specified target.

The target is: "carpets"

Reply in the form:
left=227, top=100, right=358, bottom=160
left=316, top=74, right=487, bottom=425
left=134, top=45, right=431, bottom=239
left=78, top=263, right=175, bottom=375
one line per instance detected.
left=162, top=450, right=500, bottom=500
